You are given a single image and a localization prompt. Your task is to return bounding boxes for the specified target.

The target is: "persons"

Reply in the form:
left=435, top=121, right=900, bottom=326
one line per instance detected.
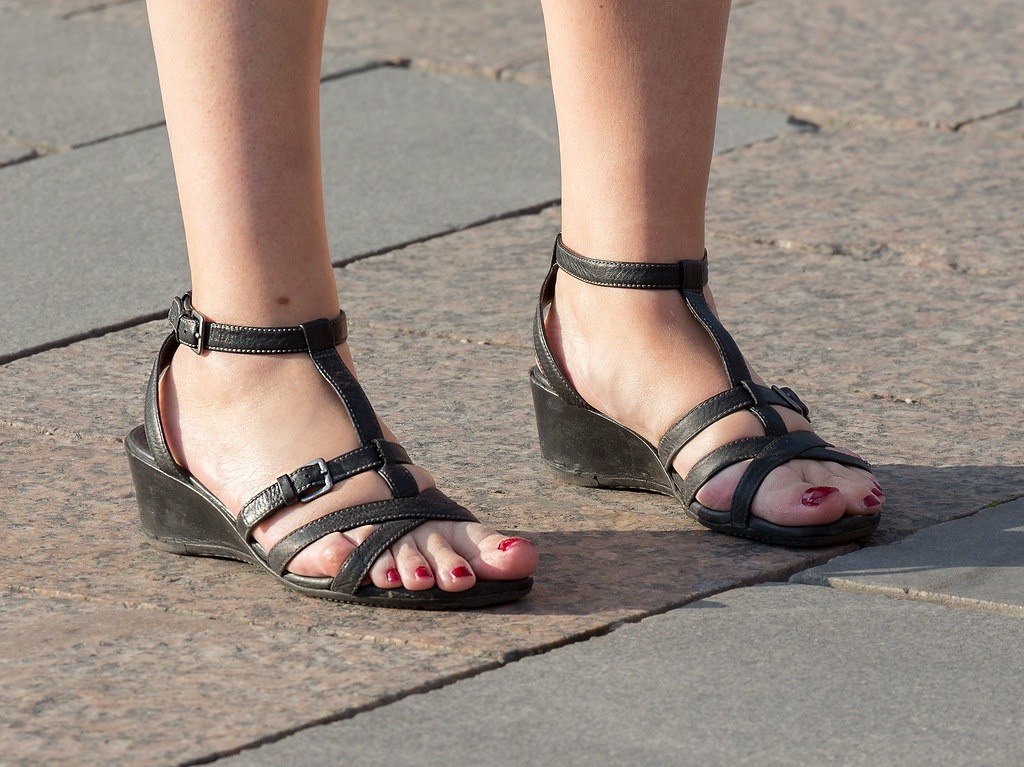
left=122, top=0, right=885, bottom=608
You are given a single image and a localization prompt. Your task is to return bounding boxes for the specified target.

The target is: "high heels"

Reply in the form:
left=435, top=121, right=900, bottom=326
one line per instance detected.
left=123, top=291, right=534, bottom=612
left=527, top=231, right=881, bottom=552
